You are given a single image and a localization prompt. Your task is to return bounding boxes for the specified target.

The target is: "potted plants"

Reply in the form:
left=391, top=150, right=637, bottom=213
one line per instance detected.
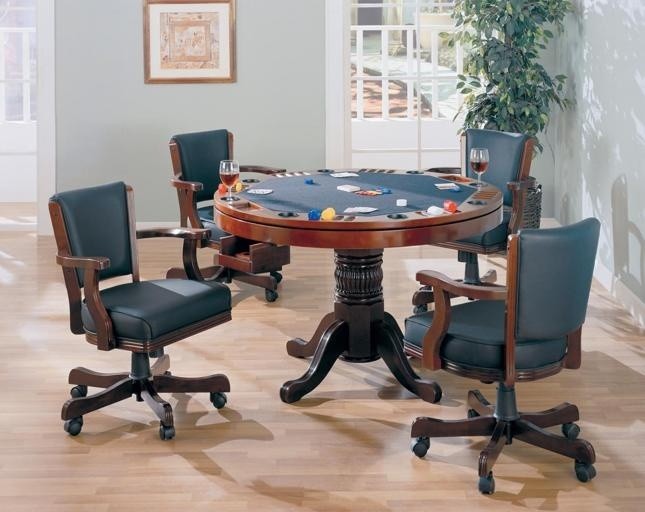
left=438, top=0, right=578, bottom=253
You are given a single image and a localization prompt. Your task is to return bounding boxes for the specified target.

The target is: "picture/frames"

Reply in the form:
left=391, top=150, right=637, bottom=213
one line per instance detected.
left=142, top=0, right=237, bottom=84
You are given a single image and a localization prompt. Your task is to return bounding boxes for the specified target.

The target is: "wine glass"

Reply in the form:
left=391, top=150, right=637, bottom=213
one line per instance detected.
left=469, top=148, right=490, bottom=188
left=219, top=159, right=240, bottom=203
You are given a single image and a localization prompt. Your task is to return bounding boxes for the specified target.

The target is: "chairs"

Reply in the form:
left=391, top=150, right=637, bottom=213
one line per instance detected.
left=47, top=181, right=232, bottom=439
left=401, top=218, right=600, bottom=493
left=412, top=129, right=542, bottom=315
left=165, top=128, right=290, bottom=301
